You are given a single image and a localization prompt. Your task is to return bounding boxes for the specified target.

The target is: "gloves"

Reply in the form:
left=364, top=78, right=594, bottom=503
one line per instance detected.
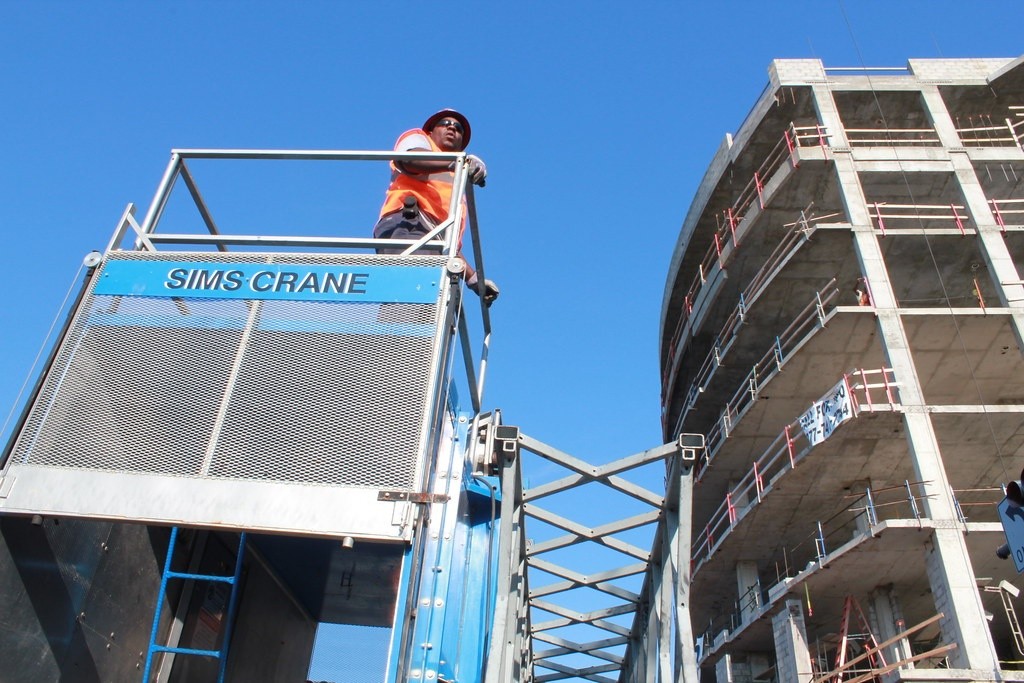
left=466, top=154, right=487, bottom=188
left=468, top=272, right=499, bottom=307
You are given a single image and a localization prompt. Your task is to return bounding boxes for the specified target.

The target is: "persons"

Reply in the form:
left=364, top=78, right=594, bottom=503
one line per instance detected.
left=372, top=109, right=499, bottom=325
left=853, top=278, right=869, bottom=306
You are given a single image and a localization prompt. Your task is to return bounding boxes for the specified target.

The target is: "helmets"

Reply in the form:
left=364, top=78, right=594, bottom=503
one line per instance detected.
left=423, top=107, right=471, bottom=150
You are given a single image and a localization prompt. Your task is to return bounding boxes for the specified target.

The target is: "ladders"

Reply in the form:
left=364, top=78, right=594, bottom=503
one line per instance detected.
left=830, top=591, right=892, bottom=683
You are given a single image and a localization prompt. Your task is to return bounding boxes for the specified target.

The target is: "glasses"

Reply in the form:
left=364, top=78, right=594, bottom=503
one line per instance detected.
left=434, top=121, right=466, bottom=134
